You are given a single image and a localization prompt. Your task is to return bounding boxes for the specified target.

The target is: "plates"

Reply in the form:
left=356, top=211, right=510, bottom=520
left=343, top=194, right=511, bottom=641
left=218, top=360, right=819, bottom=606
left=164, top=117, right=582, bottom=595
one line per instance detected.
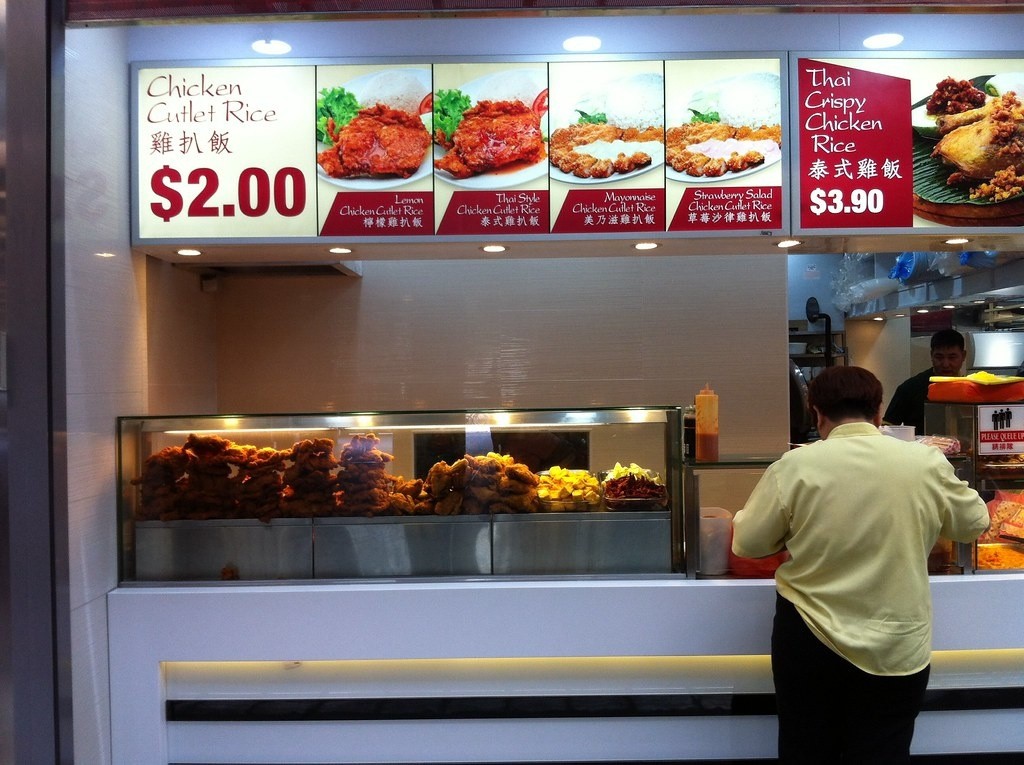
left=433, top=145, right=549, bottom=190
left=538, top=500, right=590, bottom=511
left=666, top=142, right=782, bottom=182
left=549, top=138, right=664, bottom=184
left=317, top=141, right=433, bottom=190
left=603, top=496, right=662, bottom=509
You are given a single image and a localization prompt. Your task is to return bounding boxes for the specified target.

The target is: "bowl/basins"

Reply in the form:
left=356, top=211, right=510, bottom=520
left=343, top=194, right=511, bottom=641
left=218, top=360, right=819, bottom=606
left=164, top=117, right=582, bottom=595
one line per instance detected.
left=890, top=425, right=916, bottom=441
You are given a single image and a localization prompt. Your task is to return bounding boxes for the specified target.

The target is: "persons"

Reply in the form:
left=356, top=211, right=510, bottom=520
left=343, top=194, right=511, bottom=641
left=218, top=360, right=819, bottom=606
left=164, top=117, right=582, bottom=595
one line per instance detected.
left=732, top=365, right=992, bottom=765
left=882, top=328, right=967, bottom=436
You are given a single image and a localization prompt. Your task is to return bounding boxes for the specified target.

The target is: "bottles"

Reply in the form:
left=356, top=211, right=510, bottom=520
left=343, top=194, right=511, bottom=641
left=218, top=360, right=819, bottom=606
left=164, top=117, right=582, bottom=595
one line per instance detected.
left=683, top=404, right=695, bottom=459
left=695, top=382, right=719, bottom=461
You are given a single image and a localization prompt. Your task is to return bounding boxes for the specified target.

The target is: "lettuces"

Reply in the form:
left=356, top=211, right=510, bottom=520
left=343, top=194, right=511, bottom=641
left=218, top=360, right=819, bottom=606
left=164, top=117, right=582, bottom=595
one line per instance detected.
left=434, top=88, right=472, bottom=142
left=315, top=86, right=365, bottom=145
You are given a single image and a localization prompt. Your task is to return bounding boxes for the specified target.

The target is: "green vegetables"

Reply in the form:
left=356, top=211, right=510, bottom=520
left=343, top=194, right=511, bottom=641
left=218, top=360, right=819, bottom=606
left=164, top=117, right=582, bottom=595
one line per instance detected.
left=688, top=108, right=721, bottom=123
left=574, top=109, right=608, bottom=125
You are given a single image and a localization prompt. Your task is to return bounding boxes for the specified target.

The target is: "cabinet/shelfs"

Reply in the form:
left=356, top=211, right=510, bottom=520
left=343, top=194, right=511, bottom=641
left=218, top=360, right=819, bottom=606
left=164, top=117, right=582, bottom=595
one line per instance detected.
left=922, top=400, right=1024, bottom=576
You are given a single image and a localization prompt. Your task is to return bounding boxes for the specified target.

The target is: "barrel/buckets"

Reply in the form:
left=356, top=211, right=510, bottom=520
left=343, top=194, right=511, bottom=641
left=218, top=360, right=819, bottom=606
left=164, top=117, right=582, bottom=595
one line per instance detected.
left=699, top=507, right=733, bottom=575
